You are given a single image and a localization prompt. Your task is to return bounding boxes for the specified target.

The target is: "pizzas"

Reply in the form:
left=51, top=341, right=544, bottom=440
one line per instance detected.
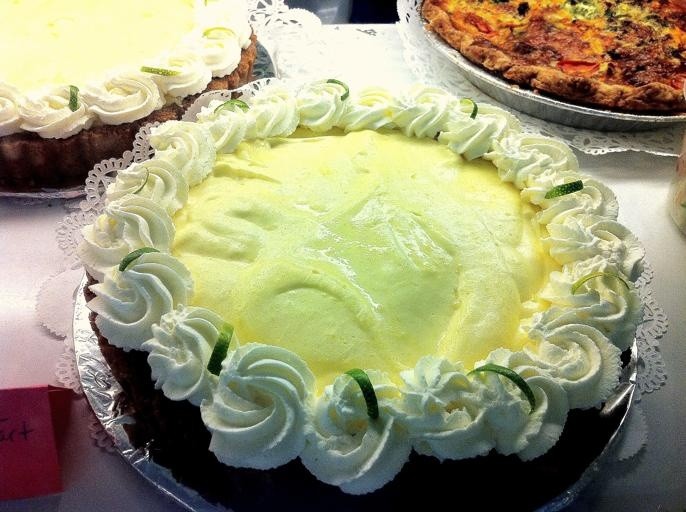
left=420, top=0, right=685, bottom=115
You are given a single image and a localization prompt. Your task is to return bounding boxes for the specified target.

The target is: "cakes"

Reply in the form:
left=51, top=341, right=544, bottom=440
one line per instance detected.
left=0, top=0, right=256, bottom=187
left=79, top=78, right=647, bottom=491
